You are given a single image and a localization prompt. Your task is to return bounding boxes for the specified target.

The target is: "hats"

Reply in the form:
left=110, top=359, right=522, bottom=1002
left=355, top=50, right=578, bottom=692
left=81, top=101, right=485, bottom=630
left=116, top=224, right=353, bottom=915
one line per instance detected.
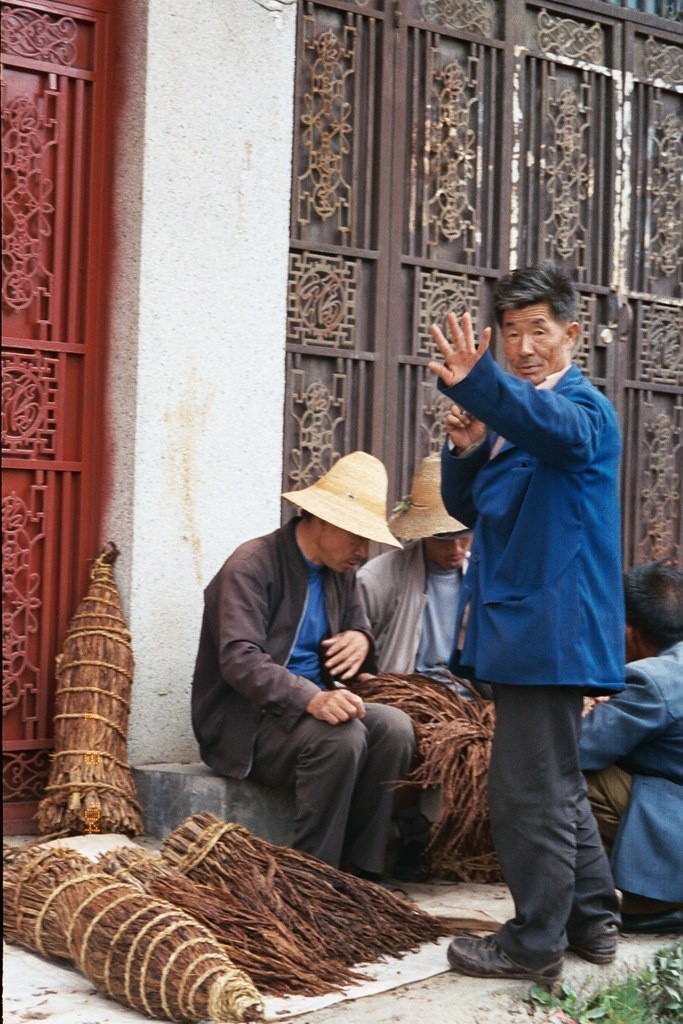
left=388, top=452, right=469, bottom=541
left=432, top=530, right=475, bottom=541
left=280, top=451, right=404, bottom=550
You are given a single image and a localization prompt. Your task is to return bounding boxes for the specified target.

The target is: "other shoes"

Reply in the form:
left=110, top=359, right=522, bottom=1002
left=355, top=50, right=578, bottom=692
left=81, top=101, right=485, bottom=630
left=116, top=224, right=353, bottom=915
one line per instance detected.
left=618, top=908, right=683, bottom=933
left=399, top=814, right=433, bottom=884
left=373, top=878, right=415, bottom=904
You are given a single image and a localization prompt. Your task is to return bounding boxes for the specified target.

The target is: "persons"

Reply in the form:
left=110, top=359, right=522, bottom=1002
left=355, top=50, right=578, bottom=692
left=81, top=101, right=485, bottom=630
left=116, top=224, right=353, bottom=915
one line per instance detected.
left=430, top=266, right=623, bottom=980
left=358, top=451, right=479, bottom=702
left=191, top=450, right=413, bottom=880
left=580, top=563, right=683, bottom=933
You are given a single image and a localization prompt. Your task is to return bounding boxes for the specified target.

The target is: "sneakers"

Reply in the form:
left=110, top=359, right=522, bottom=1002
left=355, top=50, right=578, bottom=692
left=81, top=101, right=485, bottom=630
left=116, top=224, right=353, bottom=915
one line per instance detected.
left=566, top=922, right=618, bottom=964
left=447, top=939, right=564, bottom=985
